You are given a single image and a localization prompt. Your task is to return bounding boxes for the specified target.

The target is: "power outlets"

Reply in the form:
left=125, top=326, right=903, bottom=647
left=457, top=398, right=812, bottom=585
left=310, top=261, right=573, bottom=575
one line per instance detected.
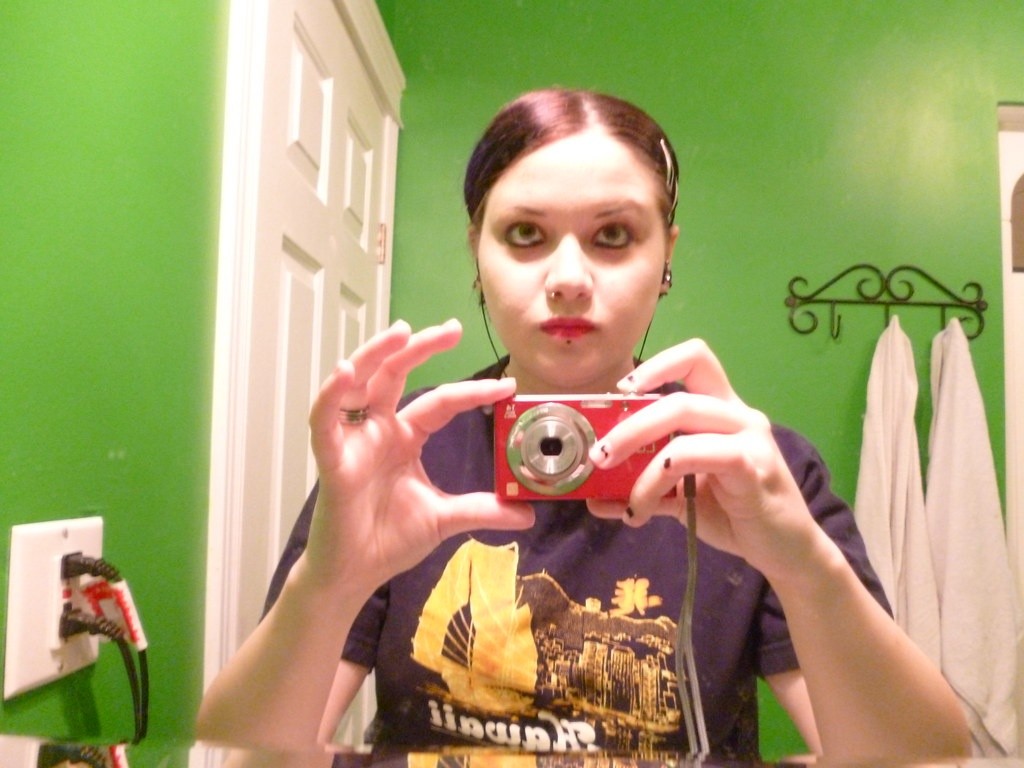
left=2, top=515, right=101, bottom=701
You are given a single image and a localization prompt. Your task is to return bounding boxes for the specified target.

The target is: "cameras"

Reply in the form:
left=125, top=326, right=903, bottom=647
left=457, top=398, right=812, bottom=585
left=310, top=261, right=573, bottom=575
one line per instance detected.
left=493, top=392, right=679, bottom=501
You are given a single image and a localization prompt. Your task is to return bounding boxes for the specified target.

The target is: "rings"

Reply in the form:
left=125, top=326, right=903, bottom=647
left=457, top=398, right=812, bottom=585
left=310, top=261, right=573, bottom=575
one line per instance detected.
left=339, top=409, right=367, bottom=423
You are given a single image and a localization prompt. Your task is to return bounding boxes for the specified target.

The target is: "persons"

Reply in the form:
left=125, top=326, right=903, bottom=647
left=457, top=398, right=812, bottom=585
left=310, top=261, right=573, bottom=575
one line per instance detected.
left=197, top=88, right=972, bottom=764
